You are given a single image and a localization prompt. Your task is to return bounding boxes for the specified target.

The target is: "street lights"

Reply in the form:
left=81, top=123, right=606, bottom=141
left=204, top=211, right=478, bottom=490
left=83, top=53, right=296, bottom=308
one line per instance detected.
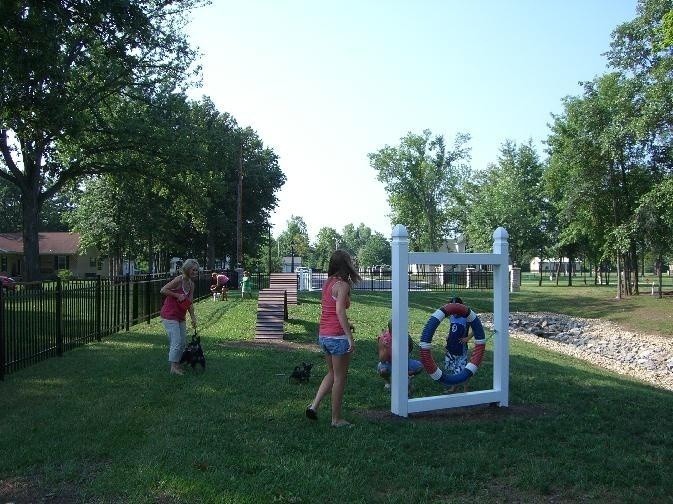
left=291, top=241, right=295, bottom=272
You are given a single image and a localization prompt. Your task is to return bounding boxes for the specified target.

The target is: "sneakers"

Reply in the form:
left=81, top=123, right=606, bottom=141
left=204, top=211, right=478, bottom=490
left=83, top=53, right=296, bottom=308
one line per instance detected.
left=384, top=384, right=391, bottom=393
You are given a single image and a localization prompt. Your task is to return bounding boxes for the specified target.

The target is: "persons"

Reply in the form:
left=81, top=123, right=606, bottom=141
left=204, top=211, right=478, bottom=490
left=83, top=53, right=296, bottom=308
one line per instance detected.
left=159, top=259, right=199, bottom=376
left=239, top=270, right=253, bottom=300
left=306, top=249, right=363, bottom=428
left=443, top=296, right=474, bottom=395
left=210, top=272, right=231, bottom=301
left=378, top=318, right=424, bottom=391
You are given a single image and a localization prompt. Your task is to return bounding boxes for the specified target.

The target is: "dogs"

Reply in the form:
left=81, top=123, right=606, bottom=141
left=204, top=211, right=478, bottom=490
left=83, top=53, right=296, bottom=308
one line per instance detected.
left=210, top=285, right=222, bottom=302
left=178, top=334, right=205, bottom=370
left=288, top=362, right=313, bottom=385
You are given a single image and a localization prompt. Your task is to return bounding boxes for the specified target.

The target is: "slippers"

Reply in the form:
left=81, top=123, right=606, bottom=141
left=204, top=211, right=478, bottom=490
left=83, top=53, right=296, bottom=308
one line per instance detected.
left=306, top=407, right=318, bottom=420
left=331, top=421, right=356, bottom=428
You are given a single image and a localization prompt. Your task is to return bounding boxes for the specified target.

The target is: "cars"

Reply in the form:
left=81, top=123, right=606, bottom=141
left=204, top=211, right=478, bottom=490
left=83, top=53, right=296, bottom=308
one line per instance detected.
left=295, top=267, right=312, bottom=272
left=0, top=276, right=17, bottom=294
